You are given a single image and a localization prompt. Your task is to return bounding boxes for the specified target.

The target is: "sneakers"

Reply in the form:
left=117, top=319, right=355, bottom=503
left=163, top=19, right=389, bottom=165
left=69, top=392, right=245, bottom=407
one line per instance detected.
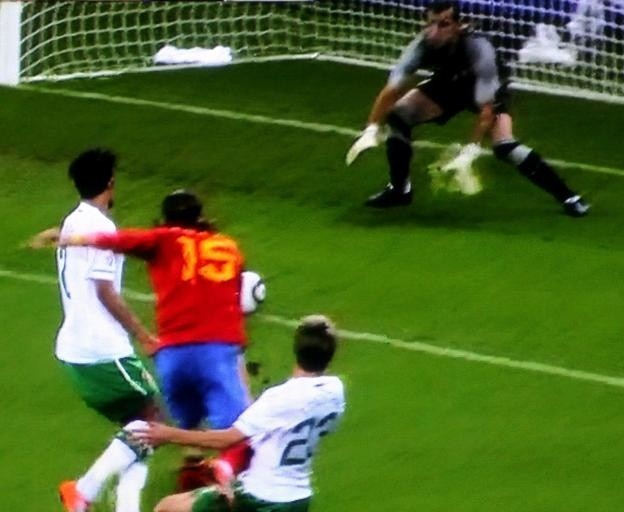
left=562, top=193, right=590, bottom=215
left=363, top=185, right=413, bottom=208
left=58, top=479, right=92, bottom=512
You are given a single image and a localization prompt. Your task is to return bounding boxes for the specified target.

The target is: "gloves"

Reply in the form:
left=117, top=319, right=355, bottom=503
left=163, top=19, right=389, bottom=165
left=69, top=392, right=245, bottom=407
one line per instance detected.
left=346, top=124, right=378, bottom=169
left=444, top=144, right=478, bottom=177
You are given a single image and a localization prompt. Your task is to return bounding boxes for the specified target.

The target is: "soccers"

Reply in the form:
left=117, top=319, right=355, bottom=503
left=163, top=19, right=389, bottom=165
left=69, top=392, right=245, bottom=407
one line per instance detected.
left=241, top=272, right=266, bottom=315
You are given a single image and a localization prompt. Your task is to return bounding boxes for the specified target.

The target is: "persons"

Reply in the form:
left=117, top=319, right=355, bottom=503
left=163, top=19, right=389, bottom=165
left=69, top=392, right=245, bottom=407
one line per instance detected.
left=22, top=186, right=256, bottom=512
left=53, top=145, right=171, bottom=512
left=125, top=312, right=347, bottom=511
left=344, top=0, right=594, bottom=218
